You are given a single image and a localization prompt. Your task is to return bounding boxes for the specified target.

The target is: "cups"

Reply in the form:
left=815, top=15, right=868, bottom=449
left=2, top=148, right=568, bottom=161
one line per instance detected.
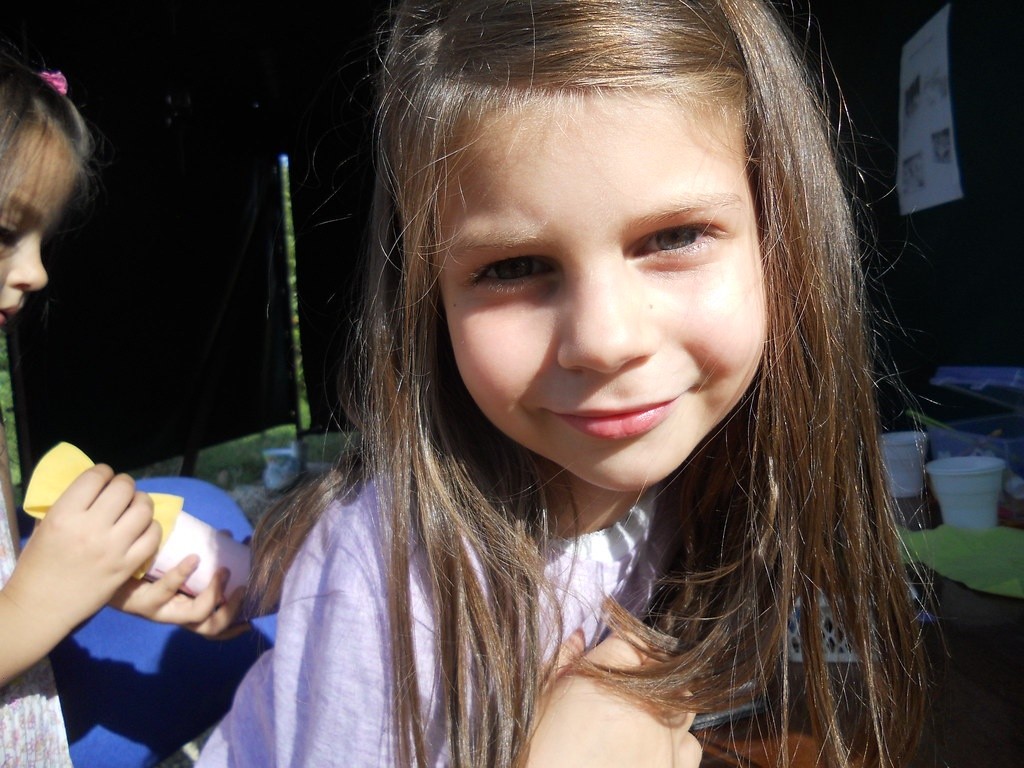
left=924, top=455, right=1007, bottom=529
left=879, top=431, right=928, bottom=498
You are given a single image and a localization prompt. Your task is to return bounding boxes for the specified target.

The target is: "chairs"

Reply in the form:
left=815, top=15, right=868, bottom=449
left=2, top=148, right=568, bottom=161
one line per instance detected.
left=20, top=477, right=278, bottom=768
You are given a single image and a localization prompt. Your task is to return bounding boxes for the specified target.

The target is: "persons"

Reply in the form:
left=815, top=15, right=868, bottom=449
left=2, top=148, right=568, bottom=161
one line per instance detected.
left=0, top=48, right=252, bottom=768
left=192, top=1, right=931, bottom=768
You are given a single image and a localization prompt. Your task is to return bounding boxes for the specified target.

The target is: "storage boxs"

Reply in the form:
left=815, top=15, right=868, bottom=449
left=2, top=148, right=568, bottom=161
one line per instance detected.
left=927, top=413, right=1024, bottom=477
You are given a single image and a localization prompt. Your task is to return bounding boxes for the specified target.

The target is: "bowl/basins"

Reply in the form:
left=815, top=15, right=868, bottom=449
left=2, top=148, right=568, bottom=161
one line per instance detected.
left=926, top=367, right=1024, bottom=478
